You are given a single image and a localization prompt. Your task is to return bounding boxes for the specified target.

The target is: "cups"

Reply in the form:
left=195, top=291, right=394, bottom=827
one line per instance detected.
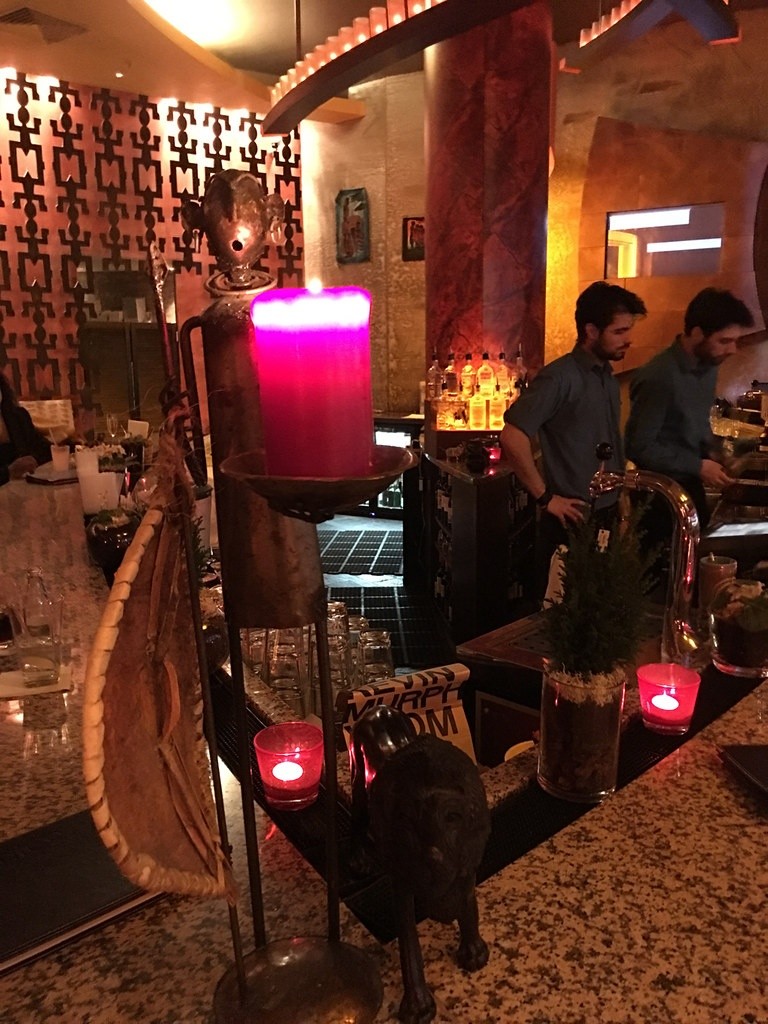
left=667, top=608, right=714, bottom=672
left=209, top=583, right=395, bottom=722
left=51, top=444, right=70, bottom=472
left=6, top=591, right=65, bottom=688
left=711, top=406, right=741, bottom=435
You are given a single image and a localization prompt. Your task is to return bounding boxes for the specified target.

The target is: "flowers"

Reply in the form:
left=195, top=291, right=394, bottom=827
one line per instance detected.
left=714, top=582, right=768, bottom=633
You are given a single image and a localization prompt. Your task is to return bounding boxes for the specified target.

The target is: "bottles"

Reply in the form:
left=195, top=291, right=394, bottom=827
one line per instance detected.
left=431, top=345, right=529, bottom=428
left=23, top=567, right=55, bottom=639
left=433, top=469, right=454, bottom=528
left=382, top=481, right=402, bottom=506
left=430, top=530, right=457, bottom=621
left=509, top=476, right=537, bottom=590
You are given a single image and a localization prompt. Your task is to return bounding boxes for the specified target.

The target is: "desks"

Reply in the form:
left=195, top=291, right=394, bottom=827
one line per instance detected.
left=457, top=599, right=712, bottom=756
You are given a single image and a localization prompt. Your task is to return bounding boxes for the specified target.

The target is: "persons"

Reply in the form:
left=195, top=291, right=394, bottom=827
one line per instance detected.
left=499, top=280, right=649, bottom=606
left=0, top=324, right=55, bottom=486
left=623, top=286, right=756, bottom=613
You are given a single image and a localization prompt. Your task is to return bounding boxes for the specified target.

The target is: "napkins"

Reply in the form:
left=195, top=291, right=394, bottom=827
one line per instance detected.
left=0, top=666, right=74, bottom=695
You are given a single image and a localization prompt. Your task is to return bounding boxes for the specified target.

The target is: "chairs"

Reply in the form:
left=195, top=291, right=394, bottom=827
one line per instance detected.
left=18, top=399, right=76, bottom=444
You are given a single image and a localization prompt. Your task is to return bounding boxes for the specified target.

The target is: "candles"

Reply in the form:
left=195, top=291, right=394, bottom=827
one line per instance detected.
left=249, top=276, right=377, bottom=477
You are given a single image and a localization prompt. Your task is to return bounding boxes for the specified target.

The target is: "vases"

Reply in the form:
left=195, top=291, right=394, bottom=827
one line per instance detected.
left=715, top=619, right=768, bottom=669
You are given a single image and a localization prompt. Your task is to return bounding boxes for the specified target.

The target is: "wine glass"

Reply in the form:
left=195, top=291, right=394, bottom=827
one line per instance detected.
left=107, top=414, right=117, bottom=445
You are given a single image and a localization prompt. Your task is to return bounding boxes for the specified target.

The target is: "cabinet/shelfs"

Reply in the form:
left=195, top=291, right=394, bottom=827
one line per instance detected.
left=416, top=445, right=552, bottom=640
left=372, top=408, right=426, bottom=519
left=79, top=320, right=180, bottom=440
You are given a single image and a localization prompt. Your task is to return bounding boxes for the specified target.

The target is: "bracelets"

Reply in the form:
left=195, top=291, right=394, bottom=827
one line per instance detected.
left=535, top=485, right=553, bottom=507
